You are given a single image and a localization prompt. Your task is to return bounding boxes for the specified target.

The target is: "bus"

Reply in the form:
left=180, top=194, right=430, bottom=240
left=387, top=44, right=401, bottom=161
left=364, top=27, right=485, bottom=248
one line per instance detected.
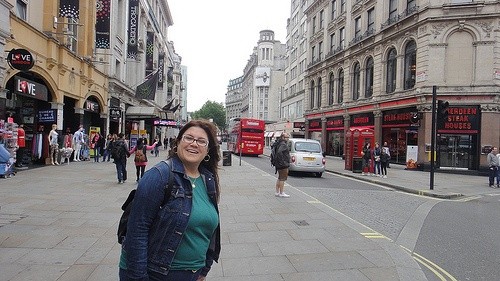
left=226, top=117, right=265, bottom=157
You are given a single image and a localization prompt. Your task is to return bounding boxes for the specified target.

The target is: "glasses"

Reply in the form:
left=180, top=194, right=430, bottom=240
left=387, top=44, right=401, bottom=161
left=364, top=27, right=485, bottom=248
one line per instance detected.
left=181, top=134, right=210, bottom=149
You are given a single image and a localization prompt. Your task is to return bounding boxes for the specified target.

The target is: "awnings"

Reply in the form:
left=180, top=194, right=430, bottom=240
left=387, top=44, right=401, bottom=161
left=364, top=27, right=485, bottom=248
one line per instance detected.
left=274, top=132, right=283, bottom=137
left=264, top=132, right=274, bottom=137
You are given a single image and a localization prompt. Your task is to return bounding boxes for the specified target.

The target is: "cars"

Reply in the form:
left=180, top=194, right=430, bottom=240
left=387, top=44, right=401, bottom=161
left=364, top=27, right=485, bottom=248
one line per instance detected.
left=286, top=138, right=326, bottom=178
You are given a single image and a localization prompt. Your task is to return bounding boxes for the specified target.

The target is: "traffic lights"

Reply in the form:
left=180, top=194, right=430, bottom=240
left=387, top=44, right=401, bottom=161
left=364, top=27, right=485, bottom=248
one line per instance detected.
left=436, top=99, right=449, bottom=130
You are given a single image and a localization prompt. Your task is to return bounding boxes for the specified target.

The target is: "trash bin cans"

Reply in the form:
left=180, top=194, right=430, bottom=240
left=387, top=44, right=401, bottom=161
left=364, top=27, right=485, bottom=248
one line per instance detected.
left=223, top=151, right=232, bottom=166
left=352, top=157, right=362, bottom=173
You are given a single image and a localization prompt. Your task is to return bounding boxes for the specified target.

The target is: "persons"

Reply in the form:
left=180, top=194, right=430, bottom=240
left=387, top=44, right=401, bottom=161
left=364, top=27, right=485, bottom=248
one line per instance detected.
left=372, top=142, right=381, bottom=176
left=273, top=140, right=278, bottom=147
left=113, top=133, right=130, bottom=183
left=141, top=135, right=147, bottom=145
left=275, top=132, right=290, bottom=197
left=271, top=138, right=274, bottom=149
left=92, top=132, right=117, bottom=163
left=381, top=148, right=390, bottom=178
left=382, top=142, right=391, bottom=168
left=164, top=136, right=168, bottom=149
left=487, top=147, right=497, bottom=188
left=48, top=124, right=59, bottom=165
left=119, top=120, right=221, bottom=281
left=154, top=136, right=160, bottom=148
left=127, top=138, right=157, bottom=181
left=362, top=141, right=371, bottom=175
left=73, top=125, right=84, bottom=161
left=153, top=140, right=160, bottom=157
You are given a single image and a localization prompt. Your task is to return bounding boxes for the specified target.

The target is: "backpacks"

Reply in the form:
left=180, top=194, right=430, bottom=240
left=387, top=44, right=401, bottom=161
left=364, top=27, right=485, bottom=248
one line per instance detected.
left=111, top=141, right=123, bottom=162
left=117, top=158, right=173, bottom=244
left=270, top=142, right=287, bottom=166
left=134, top=145, right=147, bottom=162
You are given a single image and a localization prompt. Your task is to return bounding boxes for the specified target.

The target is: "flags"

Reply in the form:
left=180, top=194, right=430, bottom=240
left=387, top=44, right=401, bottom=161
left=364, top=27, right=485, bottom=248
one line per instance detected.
left=135, top=70, right=159, bottom=100
left=163, top=100, right=179, bottom=113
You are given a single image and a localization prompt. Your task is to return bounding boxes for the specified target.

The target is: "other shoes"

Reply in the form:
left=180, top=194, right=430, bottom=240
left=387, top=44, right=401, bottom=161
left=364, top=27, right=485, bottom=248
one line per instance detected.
left=362, top=172, right=388, bottom=178
left=275, top=192, right=290, bottom=198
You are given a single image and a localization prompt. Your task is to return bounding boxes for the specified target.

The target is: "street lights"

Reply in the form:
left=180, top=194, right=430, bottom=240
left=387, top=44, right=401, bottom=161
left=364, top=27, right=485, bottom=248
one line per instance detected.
left=232, top=118, right=243, bottom=166
